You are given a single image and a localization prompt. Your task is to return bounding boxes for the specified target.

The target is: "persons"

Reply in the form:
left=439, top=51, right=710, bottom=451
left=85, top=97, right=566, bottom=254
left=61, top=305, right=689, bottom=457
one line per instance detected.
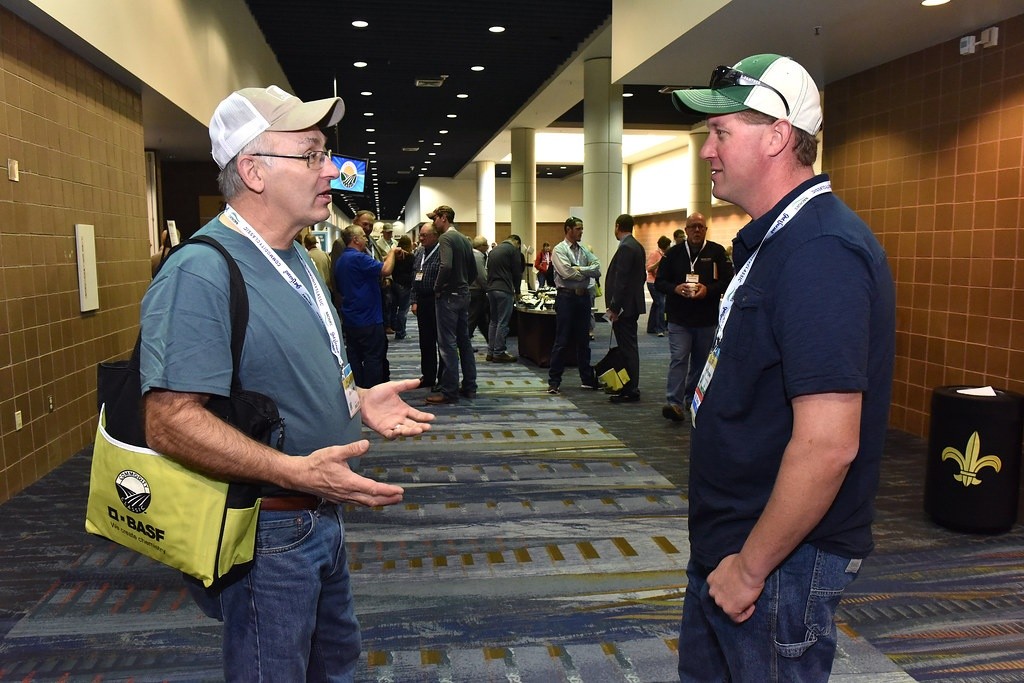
left=536, top=242, right=554, bottom=286
left=655, top=213, right=733, bottom=421
left=672, top=54, right=893, bottom=683
left=547, top=217, right=602, bottom=393
left=296, top=205, right=478, bottom=405
left=468, top=235, right=489, bottom=351
left=647, top=236, right=671, bottom=336
left=151, top=229, right=180, bottom=275
left=670, top=230, right=685, bottom=247
left=486, top=235, right=523, bottom=363
left=142, top=85, right=436, bottom=683
left=605, top=215, right=647, bottom=404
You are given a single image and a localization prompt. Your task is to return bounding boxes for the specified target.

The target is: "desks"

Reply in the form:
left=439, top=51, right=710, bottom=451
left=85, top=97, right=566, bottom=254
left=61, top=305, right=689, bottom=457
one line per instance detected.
left=515, top=290, right=598, bottom=369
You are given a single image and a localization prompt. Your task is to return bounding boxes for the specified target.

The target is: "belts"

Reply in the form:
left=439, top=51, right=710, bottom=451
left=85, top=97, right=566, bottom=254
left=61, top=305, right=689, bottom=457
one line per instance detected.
left=260, top=496, right=319, bottom=511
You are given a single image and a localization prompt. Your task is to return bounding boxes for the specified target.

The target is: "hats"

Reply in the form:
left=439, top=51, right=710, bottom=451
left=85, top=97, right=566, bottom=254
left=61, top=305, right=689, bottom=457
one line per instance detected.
left=208, top=85, right=345, bottom=172
left=426, top=205, right=455, bottom=219
left=674, top=53, right=822, bottom=137
left=383, top=223, right=394, bottom=232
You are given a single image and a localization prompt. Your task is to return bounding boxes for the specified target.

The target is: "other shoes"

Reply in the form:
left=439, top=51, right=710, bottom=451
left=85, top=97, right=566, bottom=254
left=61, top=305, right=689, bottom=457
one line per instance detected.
left=457, top=387, right=476, bottom=398
left=662, top=404, right=685, bottom=422
left=608, top=392, right=640, bottom=402
left=386, top=327, right=395, bottom=334
left=486, top=351, right=509, bottom=361
left=415, top=376, right=437, bottom=388
left=431, top=383, right=442, bottom=393
left=426, top=395, right=458, bottom=405
left=472, top=348, right=478, bottom=352
left=657, top=332, right=665, bottom=337
left=493, top=354, right=517, bottom=363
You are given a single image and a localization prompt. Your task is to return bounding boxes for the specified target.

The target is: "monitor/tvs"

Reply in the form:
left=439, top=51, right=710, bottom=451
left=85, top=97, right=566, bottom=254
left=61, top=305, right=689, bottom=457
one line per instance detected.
left=328, top=153, right=369, bottom=195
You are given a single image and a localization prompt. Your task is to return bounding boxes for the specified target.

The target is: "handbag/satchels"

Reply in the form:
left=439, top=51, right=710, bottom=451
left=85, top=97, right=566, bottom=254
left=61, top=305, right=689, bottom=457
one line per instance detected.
left=591, top=322, right=632, bottom=395
left=85, top=235, right=285, bottom=597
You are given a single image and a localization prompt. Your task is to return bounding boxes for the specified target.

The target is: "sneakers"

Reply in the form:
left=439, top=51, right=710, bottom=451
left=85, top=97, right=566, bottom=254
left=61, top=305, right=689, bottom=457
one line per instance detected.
left=547, top=384, right=560, bottom=393
left=580, top=381, right=604, bottom=389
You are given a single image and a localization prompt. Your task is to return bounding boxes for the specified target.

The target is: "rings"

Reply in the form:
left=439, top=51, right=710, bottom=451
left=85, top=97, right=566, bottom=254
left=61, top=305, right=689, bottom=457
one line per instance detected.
left=396, top=425, right=400, bottom=429
left=682, top=291, right=685, bottom=293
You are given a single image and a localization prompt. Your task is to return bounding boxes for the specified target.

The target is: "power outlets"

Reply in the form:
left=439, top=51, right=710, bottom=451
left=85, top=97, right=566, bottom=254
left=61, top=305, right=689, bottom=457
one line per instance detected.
left=48, top=396, right=53, bottom=413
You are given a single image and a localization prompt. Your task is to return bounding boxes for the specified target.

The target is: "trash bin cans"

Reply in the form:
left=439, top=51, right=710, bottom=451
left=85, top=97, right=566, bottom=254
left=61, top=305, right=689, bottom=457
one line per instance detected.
left=925, top=385, right=1024, bottom=535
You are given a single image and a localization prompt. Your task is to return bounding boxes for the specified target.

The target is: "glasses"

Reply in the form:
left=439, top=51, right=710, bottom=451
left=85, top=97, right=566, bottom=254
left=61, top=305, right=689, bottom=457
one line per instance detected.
left=249, top=149, right=331, bottom=171
left=710, top=65, right=790, bottom=116
left=418, top=233, right=434, bottom=238
